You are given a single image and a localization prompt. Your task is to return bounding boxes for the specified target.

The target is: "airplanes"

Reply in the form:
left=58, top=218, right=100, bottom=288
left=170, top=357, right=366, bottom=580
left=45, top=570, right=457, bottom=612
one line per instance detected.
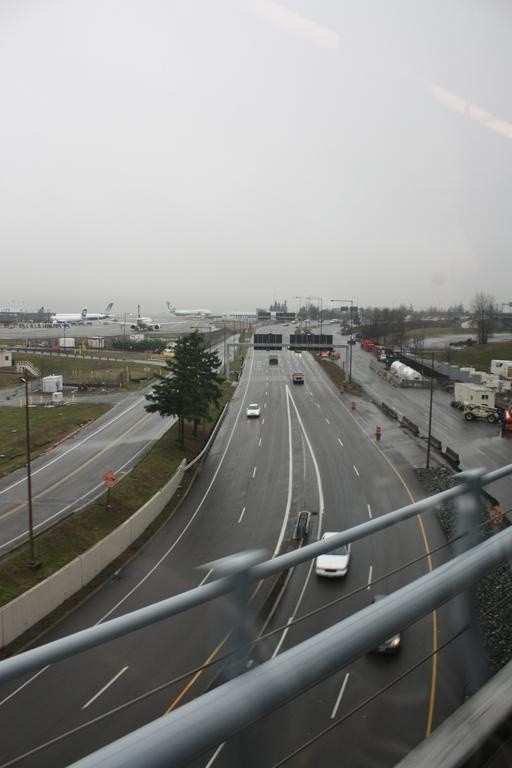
left=46, top=300, right=215, bottom=332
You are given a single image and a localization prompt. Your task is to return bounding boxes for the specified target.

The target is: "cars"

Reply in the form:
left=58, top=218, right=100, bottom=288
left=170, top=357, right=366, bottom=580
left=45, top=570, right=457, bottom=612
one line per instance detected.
left=244, top=402, right=261, bottom=418
left=268, top=355, right=279, bottom=364
left=280, top=317, right=325, bottom=329
left=316, top=531, right=351, bottom=578
left=368, top=593, right=405, bottom=654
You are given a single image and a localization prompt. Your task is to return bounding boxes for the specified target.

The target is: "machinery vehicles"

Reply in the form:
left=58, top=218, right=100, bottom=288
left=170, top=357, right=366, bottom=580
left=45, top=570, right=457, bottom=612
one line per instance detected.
left=463, top=401, right=500, bottom=423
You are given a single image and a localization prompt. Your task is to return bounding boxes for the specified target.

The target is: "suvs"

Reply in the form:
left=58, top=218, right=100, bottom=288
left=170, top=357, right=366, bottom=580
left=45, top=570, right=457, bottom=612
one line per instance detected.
left=292, top=370, right=305, bottom=384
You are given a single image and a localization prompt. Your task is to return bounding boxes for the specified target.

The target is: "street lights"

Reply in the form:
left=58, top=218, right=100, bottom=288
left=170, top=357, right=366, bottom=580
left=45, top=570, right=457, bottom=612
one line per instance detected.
left=330, top=298, right=353, bottom=384
left=307, top=297, right=322, bottom=355
left=294, top=295, right=303, bottom=334
left=17, top=358, right=42, bottom=571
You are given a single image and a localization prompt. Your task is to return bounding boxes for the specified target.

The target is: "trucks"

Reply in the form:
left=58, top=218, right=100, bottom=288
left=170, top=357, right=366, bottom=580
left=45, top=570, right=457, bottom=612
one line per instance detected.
left=348, top=332, right=394, bottom=363
left=330, top=318, right=339, bottom=324
